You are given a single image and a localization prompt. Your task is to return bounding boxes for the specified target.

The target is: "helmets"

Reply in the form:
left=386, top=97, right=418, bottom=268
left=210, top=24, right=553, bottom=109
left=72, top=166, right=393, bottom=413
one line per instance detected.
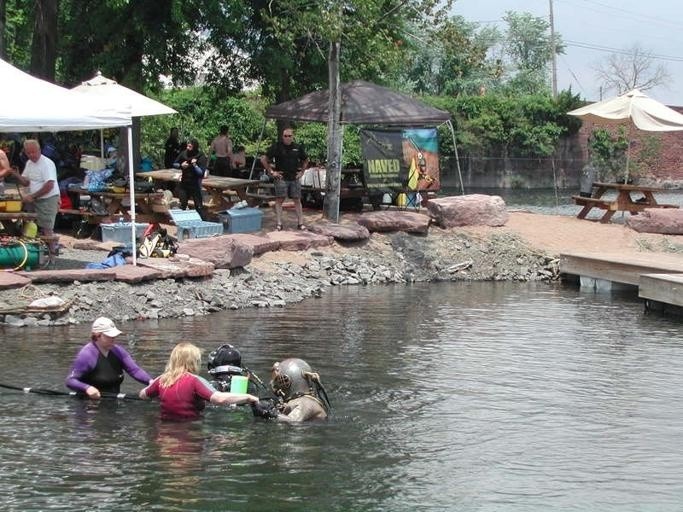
left=270, top=358, right=313, bottom=399
left=207, top=344, right=243, bottom=374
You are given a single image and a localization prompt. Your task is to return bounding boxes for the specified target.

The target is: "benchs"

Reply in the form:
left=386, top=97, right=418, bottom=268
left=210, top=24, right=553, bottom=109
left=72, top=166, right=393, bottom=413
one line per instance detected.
left=0, top=163, right=378, bottom=233
left=572, top=196, right=619, bottom=223
left=631, top=202, right=678, bottom=207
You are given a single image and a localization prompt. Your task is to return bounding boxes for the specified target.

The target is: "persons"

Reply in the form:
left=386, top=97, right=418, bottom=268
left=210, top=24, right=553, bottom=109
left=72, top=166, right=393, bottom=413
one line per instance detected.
left=254, top=358, right=331, bottom=422
left=207, top=125, right=233, bottom=177
left=206, top=344, right=259, bottom=396
left=0, top=130, right=155, bottom=271
left=138, top=342, right=260, bottom=421
left=261, top=127, right=309, bottom=232
left=164, top=127, right=180, bottom=168
left=64, top=316, right=153, bottom=401
left=172, top=139, right=205, bottom=220
left=232, top=146, right=249, bottom=178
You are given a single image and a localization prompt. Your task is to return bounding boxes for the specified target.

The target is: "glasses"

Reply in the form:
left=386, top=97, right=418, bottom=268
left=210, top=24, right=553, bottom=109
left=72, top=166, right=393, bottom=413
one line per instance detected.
left=285, top=135, right=291, bottom=137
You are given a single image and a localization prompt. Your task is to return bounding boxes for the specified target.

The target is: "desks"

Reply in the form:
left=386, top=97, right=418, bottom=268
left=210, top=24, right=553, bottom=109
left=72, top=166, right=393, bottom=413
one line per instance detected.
left=590, top=181, right=660, bottom=222
left=384, top=186, right=437, bottom=211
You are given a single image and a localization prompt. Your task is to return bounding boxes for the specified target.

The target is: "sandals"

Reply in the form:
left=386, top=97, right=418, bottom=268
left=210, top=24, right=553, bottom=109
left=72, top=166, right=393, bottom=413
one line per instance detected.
left=276, top=224, right=282, bottom=231
left=297, top=224, right=307, bottom=230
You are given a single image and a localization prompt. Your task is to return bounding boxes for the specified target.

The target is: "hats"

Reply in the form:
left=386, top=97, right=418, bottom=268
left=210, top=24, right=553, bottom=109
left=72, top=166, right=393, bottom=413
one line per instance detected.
left=92, top=317, right=122, bottom=338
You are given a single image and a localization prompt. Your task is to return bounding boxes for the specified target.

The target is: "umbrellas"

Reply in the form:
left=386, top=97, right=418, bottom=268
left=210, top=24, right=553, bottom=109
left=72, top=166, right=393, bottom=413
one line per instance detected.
left=565, top=84, right=683, bottom=185
left=70, top=71, right=178, bottom=159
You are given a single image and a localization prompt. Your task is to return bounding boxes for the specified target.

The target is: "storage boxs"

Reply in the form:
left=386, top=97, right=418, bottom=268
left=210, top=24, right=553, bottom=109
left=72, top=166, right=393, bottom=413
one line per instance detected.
left=168, top=208, right=223, bottom=242
left=99, top=223, right=148, bottom=243
